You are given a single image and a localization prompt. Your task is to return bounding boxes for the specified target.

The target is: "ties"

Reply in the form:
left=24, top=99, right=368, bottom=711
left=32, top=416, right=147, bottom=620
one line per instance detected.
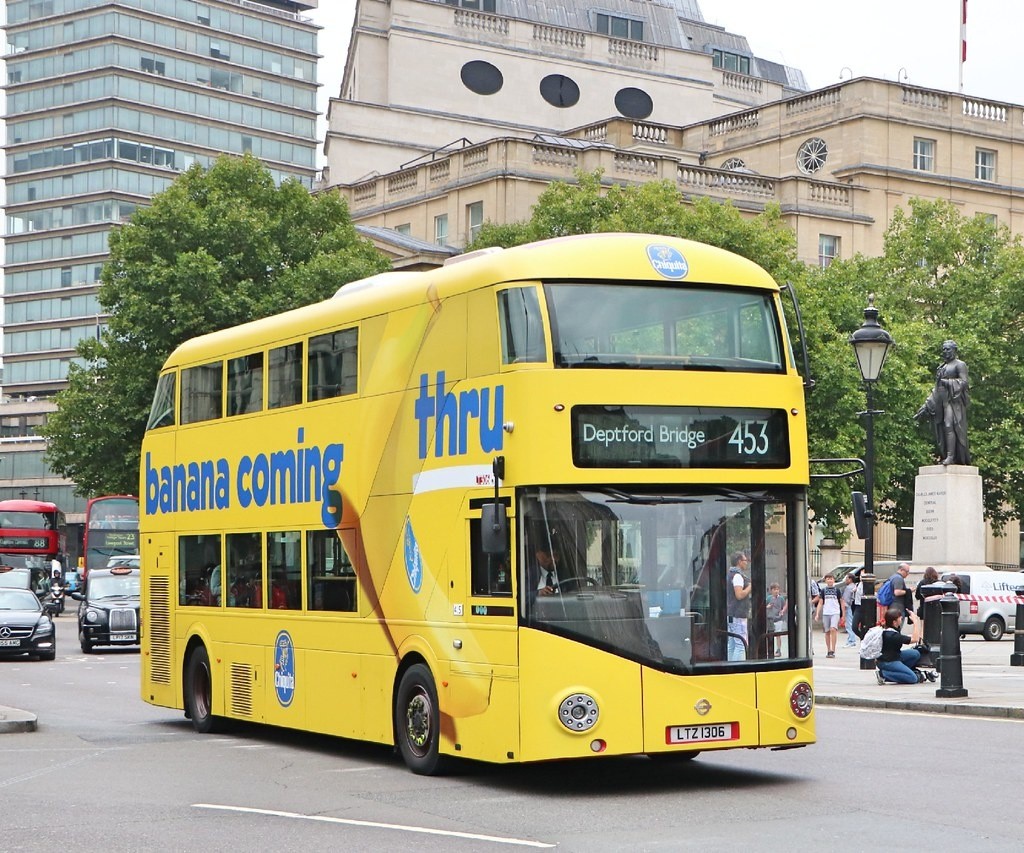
left=544, top=571, right=556, bottom=593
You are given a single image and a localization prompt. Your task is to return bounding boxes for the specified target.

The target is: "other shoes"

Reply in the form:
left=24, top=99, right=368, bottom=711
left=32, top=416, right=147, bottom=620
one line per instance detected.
left=775, top=651, right=781, bottom=657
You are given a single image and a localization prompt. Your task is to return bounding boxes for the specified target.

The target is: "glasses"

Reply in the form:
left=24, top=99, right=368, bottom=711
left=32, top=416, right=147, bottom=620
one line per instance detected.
left=903, top=568, right=909, bottom=574
left=741, top=559, right=747, bottom=561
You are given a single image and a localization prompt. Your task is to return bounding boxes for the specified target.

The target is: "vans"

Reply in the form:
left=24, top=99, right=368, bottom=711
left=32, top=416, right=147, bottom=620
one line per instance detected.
left=940, top=572, right=1024, bottom=641
left=815, top=561, right=911, bottom=605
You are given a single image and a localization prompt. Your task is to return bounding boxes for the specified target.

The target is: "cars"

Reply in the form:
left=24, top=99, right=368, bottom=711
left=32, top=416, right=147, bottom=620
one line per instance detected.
left=105, top=554, right=140, bottom=569
left=0, top=567, right=51, bottom=602
left=1, top=588, right=59, bottom=660
left=70, top=568, right=140, bottom=652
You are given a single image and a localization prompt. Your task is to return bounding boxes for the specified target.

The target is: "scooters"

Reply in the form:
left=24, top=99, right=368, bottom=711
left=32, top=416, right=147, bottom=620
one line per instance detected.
left=46, top=586, right=65, bottom=617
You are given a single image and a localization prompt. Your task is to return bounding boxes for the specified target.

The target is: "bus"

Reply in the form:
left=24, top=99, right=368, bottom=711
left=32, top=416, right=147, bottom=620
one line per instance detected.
left=0, top=499, right=67, bottom=581
left=66, top=495, right=139, bottom=594
left=135, top=228, right=873, bottom=776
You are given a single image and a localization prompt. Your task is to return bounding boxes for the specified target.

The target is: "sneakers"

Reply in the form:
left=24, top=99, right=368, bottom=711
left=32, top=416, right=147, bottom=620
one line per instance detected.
left=843, top=640, right=856, bottom=648
left=827, top=651, right=835, bottom=658
left=875, top=670, right=885, bottom=685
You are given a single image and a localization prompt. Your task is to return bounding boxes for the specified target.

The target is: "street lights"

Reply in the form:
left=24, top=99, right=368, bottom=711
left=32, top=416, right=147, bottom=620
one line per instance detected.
left=844, top=291, right=896, bottom=669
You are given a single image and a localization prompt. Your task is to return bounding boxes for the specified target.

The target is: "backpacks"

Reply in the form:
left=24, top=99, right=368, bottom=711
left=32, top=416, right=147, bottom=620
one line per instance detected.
left=877, top=581, right=893, bottom=605
left=860, top=626, right=882, bottom=660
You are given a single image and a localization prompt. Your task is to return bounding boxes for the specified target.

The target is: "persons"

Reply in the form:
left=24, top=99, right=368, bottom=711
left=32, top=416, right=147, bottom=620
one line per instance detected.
left=726, top=551, right=752, bottom=662
left=912, top=340, right=972, bottom=465
left=50, top=570, right=66, bottom=597
left=747, top=563, right=962, bottom=685
left=210, top=564, right=236, bottom=607
left=525, top=533, right=580, bottom=602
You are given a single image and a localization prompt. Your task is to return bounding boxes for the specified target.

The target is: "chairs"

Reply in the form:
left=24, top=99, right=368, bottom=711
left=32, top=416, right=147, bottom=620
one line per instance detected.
left=255, top=584, right=287, bottom=609
